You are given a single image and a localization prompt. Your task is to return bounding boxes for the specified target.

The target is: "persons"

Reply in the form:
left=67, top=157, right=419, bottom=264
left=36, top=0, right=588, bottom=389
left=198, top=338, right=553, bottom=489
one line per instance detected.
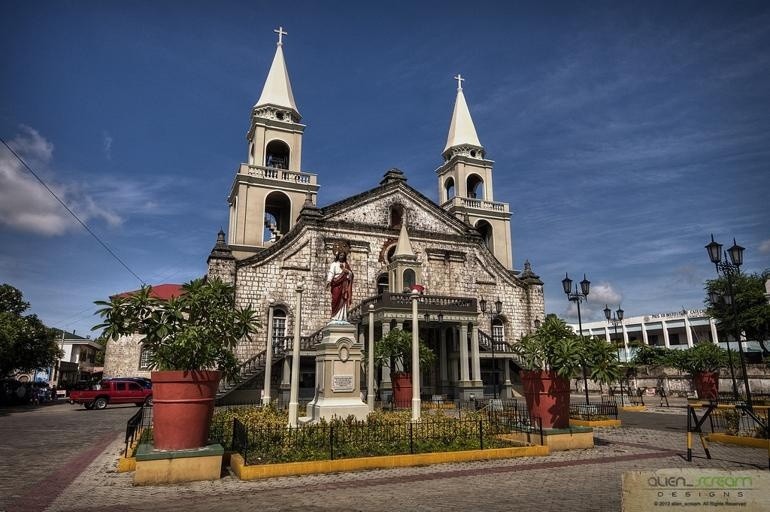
left=50, top=383, right=57, bottom=400
left=324, top=252, right=354, bottom=325
left=32, top=384, right=40, bottom=407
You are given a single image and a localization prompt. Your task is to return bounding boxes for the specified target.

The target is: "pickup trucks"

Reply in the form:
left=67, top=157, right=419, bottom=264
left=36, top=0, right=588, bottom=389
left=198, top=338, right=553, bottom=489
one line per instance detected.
left=68, top=380, right=153, bottom=410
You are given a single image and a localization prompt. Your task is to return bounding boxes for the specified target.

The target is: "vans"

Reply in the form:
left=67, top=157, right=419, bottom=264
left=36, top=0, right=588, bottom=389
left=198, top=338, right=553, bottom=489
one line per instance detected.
left=108, top=378, right=152, bottom=390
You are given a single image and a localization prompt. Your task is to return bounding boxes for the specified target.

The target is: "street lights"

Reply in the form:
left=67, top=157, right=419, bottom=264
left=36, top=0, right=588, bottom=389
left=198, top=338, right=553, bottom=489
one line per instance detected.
left=479, top=293, right=502, bottom=400
left=560, top=271, right=590, bottom=407
left=709, top=285, right=742, bottom=401
left=704, top=229, right=757, bottom=412
left=602, top=302, right=625, bottom=408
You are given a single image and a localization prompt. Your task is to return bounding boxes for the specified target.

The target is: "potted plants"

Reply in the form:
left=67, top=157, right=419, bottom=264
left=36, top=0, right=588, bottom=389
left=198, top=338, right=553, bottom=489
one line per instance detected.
left=91, top=275, right=261, bottom=450
left=361, top=327, right=438, bottom=408
left=513, top=317, right=620, bottom=428
left=629, top=341, right=740, bottom=399
